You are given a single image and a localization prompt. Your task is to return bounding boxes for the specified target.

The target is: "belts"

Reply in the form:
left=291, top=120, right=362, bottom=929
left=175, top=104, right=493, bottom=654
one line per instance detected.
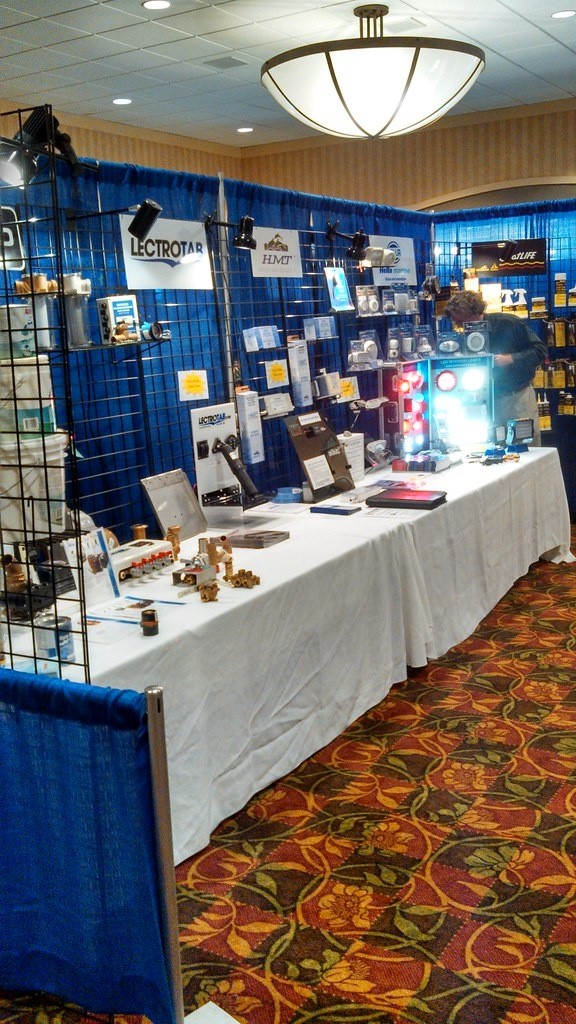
left=494, top=383, right=530, bottom=395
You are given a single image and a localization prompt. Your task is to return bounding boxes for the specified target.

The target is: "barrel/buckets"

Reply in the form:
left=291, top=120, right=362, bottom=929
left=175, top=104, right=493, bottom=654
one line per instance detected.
left=34, top=616, right=75, bottom=667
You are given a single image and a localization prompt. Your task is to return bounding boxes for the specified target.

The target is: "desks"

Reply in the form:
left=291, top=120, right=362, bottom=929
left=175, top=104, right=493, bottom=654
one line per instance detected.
left=0, top=448, right=576, bottom=867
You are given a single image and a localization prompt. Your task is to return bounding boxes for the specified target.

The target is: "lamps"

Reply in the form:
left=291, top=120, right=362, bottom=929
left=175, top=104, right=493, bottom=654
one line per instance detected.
left=202, top=209, right=257, bottom=250
left=65, top=196, right=163, bottom=243
left=259, top=5, right=485, bottom=141
left=0, top=106, right=88, bottom=191
left=325, top=219, right=369, bottom=261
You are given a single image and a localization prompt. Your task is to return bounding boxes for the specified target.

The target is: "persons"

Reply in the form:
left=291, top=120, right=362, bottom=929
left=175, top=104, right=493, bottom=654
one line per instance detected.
left=443, top=290, right=548, bottom=448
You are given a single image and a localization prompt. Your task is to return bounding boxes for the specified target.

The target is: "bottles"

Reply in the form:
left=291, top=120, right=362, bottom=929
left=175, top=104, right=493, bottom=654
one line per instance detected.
left=558, top=391, right=565, bottom=414
left=537, top=394, right=544, bottom=431
left=543, top=393, right=551, bottom=429
left=564, top=394, right=574, bottom=414
